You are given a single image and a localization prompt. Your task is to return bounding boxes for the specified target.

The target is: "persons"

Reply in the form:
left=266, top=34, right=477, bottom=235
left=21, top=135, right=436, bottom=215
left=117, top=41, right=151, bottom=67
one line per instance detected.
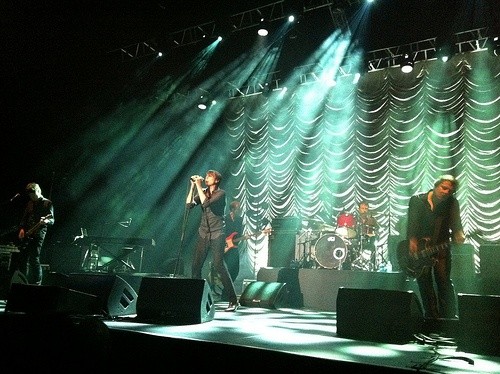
left=350, top=201, right=379, bottom=272
left=219, top=201, right=243, bottom=302
left=186, top=169, right=241, bottom=311
left=18, top=183, right=55, bottom=285
left=407, top=174, right=465, bottom=330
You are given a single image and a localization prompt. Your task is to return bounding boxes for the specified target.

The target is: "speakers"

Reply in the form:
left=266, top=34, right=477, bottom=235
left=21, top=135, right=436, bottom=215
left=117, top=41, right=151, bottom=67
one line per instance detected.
left=238, top=281, right=289, bottom=309
left=6, top=270, right=139, bottom=318
left=336, top=286, right=429, bottom=345
left=136, top=277, right=215, bottom=324
left=456, top=293, right=500, bottom=357
left=479, top=243, right=500, bottom=280
left=268, top=230, right=297, bottom=267
left=451, top=243, right=476, bottom=281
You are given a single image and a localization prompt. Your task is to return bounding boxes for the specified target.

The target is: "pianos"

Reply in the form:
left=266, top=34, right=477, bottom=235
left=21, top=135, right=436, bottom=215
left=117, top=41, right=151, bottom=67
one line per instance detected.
left=64, top=236, right=157, bottom=273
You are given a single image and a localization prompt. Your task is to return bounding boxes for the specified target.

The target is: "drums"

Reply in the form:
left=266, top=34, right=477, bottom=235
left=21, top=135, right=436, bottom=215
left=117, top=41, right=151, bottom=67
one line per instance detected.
left=314, top=234, right=347, bottom=269
left=358, top=225, right=376, bottom=237
left=335, top=213, right=358, bottom=239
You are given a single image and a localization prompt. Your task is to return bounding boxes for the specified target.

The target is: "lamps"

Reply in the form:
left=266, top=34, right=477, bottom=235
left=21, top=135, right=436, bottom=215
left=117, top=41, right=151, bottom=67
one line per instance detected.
left=158, top=47, right=169, bottom=57
left=197, top=95, right=209, bottom=110
left=435, top=34, right=455, bottom=64
left=262, top=83, right=272, bottom=98
left=217, top=33, right=225, bottom=42
left=400, top=49, right=414, bottom=74
left=257, top=15, right=269, bottom=36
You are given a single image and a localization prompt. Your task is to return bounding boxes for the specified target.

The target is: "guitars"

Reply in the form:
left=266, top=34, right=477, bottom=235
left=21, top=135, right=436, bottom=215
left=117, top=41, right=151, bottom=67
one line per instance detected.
left=224, top=227, right=274, bottom=253
left=397, top=229, right=484, bottom=279
left=12, top=213, right=53, bottom=249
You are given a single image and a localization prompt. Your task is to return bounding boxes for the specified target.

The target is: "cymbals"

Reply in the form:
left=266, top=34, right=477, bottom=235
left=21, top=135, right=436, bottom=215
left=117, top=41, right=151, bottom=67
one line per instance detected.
left=303, top=219, right=322, bottom=222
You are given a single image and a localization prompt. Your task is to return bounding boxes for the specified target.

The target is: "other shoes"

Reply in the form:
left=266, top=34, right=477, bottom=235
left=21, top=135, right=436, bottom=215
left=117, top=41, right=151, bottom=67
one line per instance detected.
left=224, top=302, right=238, bottom=312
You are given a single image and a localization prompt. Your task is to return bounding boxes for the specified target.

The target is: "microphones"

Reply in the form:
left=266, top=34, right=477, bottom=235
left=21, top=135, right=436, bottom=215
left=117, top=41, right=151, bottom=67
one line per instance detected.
left=189, top=177, right=204, bottom=182
left=10, top=193, right=20, bottom=202
left=129, top=218, right=132, bottom=226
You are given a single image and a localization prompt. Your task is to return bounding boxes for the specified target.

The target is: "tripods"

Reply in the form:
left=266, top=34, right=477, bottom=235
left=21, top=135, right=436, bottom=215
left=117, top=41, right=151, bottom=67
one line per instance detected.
left=298, top=238, right=319, bottom=269
left=351, top=227, right=373, bottom=271
